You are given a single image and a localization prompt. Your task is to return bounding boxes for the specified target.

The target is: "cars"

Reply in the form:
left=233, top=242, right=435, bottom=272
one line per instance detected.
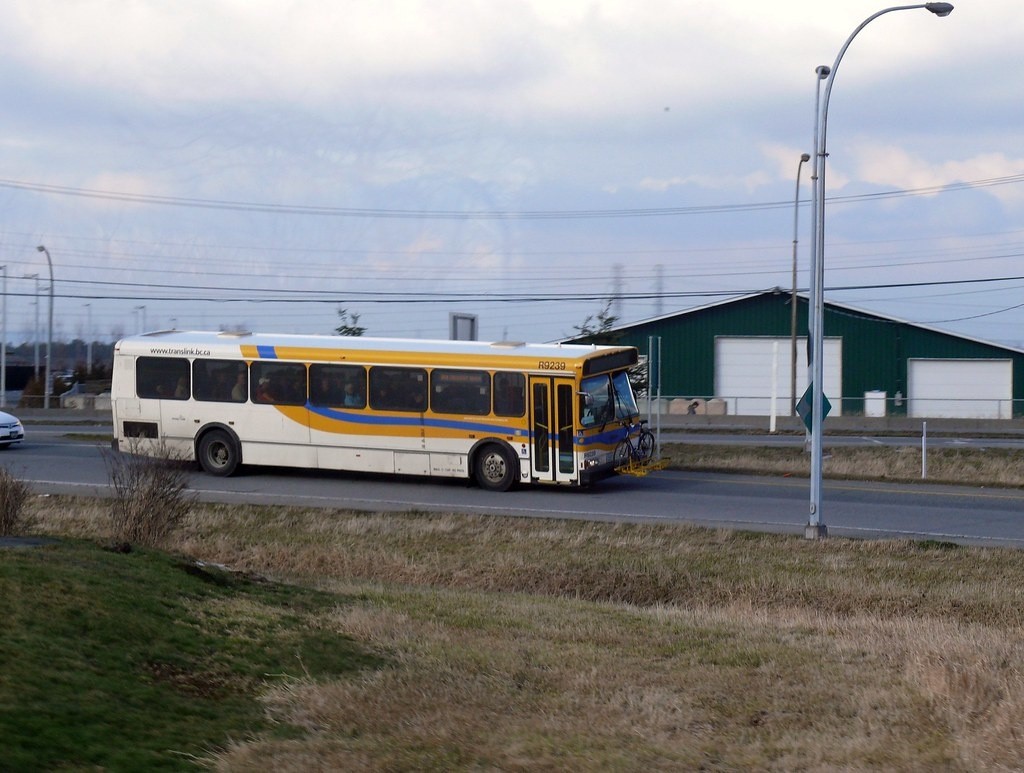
left=0, top=410, right=25, bottom=450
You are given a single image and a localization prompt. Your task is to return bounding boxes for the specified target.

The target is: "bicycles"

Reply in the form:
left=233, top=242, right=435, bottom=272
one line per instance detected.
left=613, top=418, right=655, bottom=471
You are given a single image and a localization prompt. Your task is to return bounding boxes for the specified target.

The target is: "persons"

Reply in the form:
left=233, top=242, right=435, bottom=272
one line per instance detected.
left=687, top=401, right=698, bottom=414
left=169, top=367, right=524, bottom=416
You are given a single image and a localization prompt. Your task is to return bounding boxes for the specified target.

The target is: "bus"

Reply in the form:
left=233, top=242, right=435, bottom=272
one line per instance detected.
left=109, top=327, right=670, bottom=493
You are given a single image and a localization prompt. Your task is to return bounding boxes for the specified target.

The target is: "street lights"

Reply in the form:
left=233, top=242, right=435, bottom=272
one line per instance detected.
left=789, top=153, right=812, bottom=417
left=37, top=245, right=55, bottom=410
left=800, top=1, right=956, bottom=541
